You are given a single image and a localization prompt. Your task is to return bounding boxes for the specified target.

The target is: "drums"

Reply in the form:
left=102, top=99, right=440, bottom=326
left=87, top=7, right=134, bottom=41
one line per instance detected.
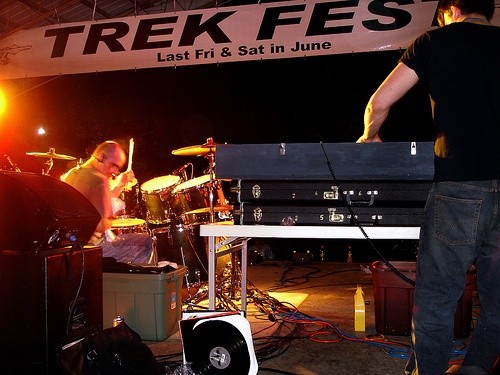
left=108, top=176, right=140, bottom=217
left=153, top=222, right=208, bottom=288
left=147, top=234, right=233, bottom=301
left=111, top=219, right=148, bottom=238
left=175, top=173, right=235, bottom=228
left=141, top=174, right=186, bottom=225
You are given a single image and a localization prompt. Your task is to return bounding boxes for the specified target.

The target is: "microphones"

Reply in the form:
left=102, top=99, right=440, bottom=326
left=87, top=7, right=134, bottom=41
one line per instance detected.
left=172, top=162, right=189, bottom=176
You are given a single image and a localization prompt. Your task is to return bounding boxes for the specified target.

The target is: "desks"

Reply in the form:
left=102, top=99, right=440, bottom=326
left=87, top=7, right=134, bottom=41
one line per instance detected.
left=200, top=221, right=421, bottom=311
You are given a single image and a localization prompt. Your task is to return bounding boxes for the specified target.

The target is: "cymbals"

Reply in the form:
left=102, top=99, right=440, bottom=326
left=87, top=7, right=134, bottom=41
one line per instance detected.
left=184, top=205, right=235, bottom=216
left=172, top=143, right=221, bottom=157
left=25, top=152, right=77, bottom=160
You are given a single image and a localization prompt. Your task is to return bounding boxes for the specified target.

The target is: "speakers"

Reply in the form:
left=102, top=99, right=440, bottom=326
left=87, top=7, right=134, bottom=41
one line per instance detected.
left=79, top=324, right=168, bottom=375
left=1, top=243, right=104, bottom=368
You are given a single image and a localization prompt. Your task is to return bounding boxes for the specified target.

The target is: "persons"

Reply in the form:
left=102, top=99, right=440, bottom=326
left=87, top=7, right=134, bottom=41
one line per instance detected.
left=355, top=0, right=500, bottom=375
left=61, top=140, right=154, bottom=265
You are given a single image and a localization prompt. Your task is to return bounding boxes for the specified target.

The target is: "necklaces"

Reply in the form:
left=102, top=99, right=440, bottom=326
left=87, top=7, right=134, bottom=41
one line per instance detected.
left=463, top=18, right=485, bottom=22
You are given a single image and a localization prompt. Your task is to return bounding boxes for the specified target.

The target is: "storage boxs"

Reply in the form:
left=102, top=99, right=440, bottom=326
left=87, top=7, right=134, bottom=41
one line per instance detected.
left=102, top=264, right=187, bottom=341
left=372, top=261, right=472, bottom=340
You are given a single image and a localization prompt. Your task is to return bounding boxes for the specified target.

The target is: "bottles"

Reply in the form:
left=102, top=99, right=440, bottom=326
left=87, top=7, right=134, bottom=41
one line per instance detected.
left=347, top=246, right=352, bottom=265
left=320, top=246, right=325, bottom=263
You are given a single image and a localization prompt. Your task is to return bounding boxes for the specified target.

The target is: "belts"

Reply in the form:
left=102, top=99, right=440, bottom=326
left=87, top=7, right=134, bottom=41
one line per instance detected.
left=442, top=173, right=500, bottom=182
